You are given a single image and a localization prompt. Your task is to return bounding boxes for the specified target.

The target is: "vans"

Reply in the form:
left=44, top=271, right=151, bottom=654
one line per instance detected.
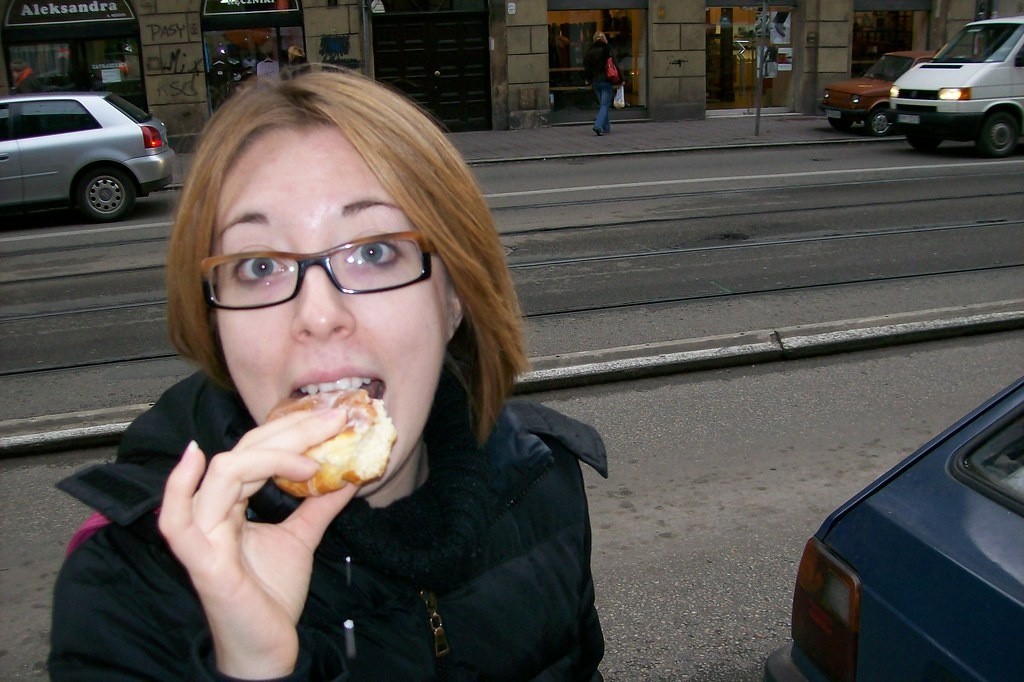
left=885, top=15, right=1024, bottom=159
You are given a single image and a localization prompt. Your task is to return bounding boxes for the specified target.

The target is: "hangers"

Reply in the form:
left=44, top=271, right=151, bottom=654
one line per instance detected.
left=212, top=50, right=277, bottom=65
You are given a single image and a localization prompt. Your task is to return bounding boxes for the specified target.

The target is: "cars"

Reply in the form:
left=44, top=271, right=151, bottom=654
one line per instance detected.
left=761, top=376, right=1024, bottom=682
left=819, top=49, right=983, bottom=137
left=0, top=88, right=177, bottom=223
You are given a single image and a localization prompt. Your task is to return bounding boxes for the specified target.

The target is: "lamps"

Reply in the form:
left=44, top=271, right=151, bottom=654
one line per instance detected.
left=371, top=0, right=385, bottom=13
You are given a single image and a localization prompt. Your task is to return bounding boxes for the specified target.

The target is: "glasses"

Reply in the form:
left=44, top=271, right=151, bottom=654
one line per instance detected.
left=200, top=232, right=438, bottom=310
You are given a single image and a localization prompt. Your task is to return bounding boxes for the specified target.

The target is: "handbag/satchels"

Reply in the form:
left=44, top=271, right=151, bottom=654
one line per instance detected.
left=606, top=49, right=619, bottom=83
left=613, top=86, right=625, bottom=109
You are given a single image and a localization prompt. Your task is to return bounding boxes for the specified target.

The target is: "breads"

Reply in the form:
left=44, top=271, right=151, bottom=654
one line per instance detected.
left=263, top=390, right=396, bottom=495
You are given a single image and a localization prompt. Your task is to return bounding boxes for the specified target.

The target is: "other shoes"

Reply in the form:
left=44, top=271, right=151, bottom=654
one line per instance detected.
left=593, top=127, right=603, bottom=136
left=604, top=125, right=610, bottom=133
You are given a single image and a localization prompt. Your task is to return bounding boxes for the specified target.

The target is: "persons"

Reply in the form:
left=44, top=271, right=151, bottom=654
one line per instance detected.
left=584, top=32, right=626, bottom=136
left=281, top=45, right=310, bottom=79
left=47, top=64, right=609, bottom=682
left=10, top=58, right=40, bottom=94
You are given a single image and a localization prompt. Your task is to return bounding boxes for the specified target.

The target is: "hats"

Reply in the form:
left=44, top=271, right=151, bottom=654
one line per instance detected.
left=10, top=58, right=26, bottom=70
